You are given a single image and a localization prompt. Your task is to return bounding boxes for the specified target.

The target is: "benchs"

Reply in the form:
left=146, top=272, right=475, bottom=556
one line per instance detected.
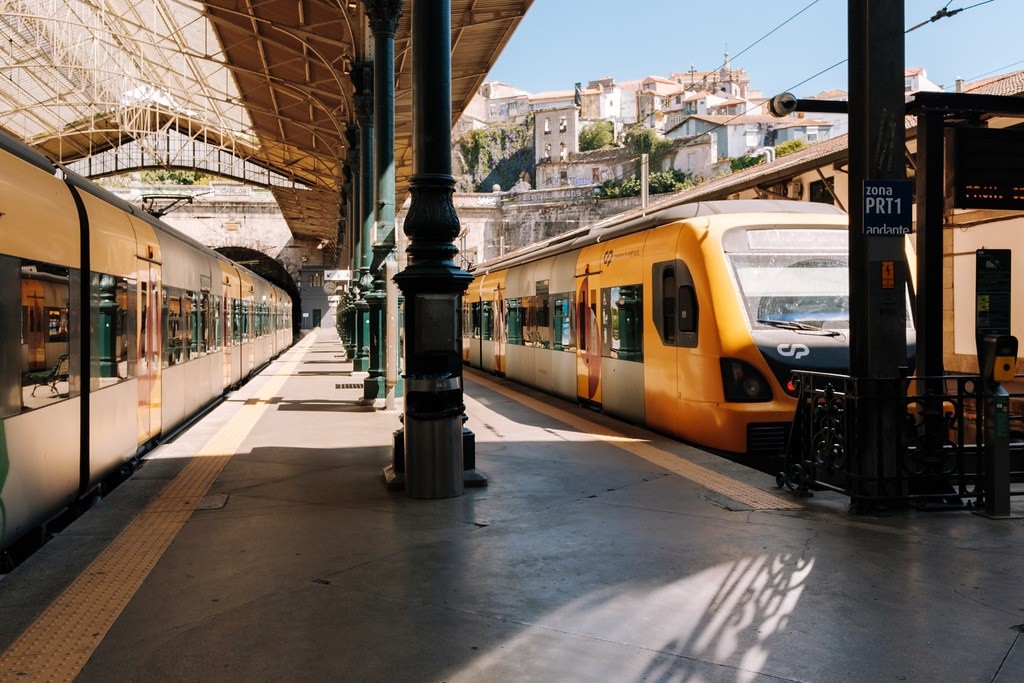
left=26, top=353, right=68, bottom=399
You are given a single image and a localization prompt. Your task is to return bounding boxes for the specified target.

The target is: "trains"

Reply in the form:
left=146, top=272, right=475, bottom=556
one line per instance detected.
left=459, top=198, right=926, bottom=464
left=0, top=121, right=305, bottom=560
